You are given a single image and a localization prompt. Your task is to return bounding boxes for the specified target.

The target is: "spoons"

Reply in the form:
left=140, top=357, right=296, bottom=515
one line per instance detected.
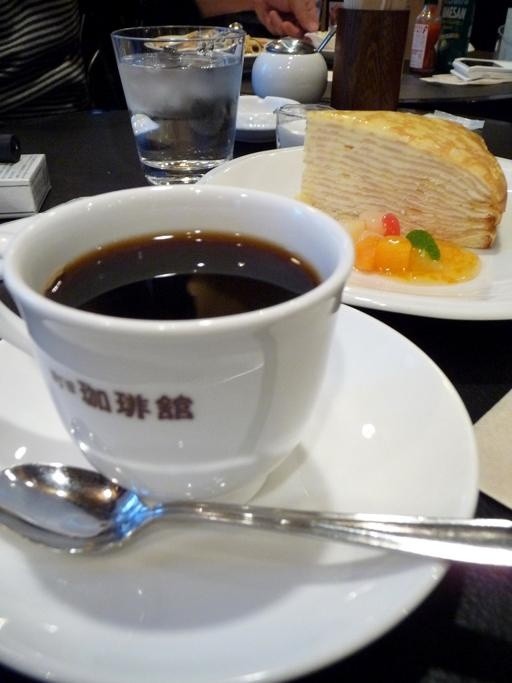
left=0, top=461, right=512, bottom=568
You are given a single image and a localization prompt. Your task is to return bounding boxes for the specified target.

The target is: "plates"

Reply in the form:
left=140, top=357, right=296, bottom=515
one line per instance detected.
left=1, top=301, right=482, bottom=682
left=190, top=144, right=510, bottom=322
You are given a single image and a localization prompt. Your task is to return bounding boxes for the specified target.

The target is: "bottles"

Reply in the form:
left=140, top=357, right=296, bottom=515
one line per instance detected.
left=408, top=0, right=442, bottom=75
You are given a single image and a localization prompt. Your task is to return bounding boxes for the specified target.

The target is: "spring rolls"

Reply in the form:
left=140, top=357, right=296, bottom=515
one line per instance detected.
left=244, top=39, right=261, bottom=55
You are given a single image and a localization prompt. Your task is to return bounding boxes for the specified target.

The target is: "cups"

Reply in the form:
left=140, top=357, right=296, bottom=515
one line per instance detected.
left=110, top=22, right=247, bottom=186
left=272, top=100, right=338, bottom=147
left=3, top=181, right=358, bottom=507
left=332, top=4, right=409, bottom=113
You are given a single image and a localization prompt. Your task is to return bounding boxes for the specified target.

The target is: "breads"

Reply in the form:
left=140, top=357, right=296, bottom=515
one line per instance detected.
left=299, top=111, right=508, bottom=250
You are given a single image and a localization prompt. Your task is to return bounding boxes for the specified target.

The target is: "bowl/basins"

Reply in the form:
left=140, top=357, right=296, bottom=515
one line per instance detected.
left=301, top=29, right=335, bottom=59
left=251, top=51, right=328, bottom=102
left=140, top=33, right=273, bottom=75
left=234, top=93, right=303, bottom=143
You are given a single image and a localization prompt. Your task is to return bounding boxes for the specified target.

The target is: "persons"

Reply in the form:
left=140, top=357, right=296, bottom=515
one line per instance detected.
left=0, top=1, right=320, bottom=125
